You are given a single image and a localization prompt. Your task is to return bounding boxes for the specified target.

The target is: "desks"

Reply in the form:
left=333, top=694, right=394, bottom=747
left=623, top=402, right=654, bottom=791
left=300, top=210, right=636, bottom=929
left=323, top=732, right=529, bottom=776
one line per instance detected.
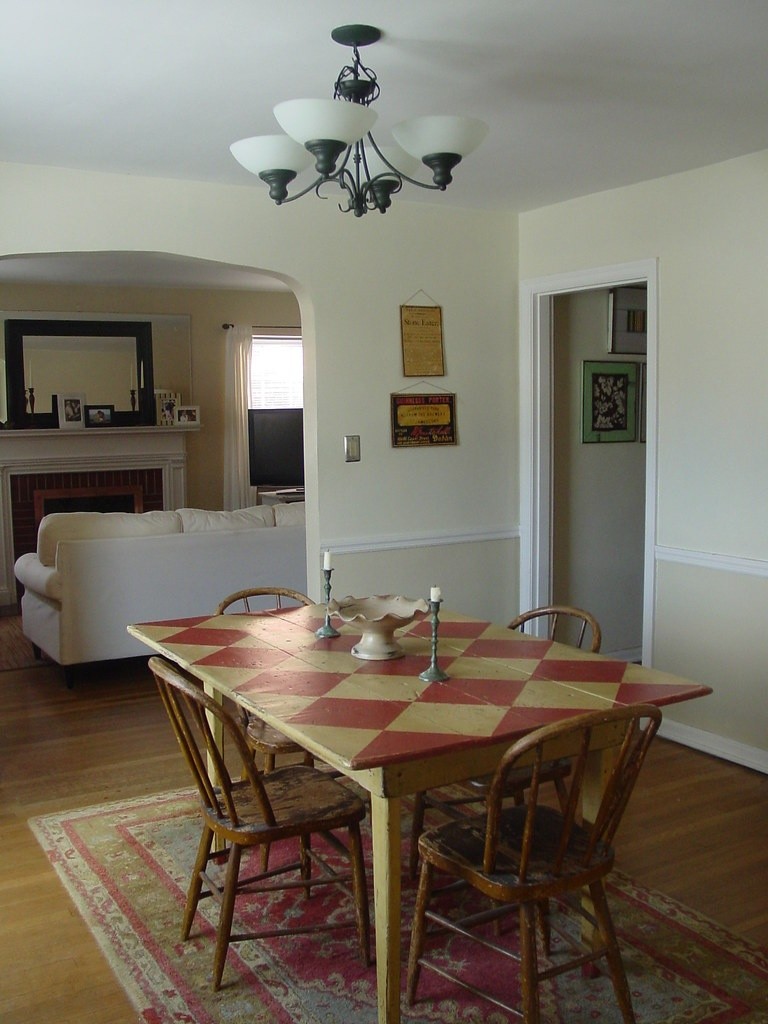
left=127, top=602, right=713, bottom=1023
left=259, top=490, right=305, bottom=506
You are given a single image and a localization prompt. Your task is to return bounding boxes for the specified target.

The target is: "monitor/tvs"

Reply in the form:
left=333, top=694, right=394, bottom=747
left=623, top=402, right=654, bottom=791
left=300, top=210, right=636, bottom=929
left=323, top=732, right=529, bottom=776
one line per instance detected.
left=249, top=408, right=305, bottom=495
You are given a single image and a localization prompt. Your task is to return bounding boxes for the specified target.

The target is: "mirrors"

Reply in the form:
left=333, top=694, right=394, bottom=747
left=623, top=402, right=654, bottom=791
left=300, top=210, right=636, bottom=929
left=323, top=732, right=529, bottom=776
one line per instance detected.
left=4, top=318, right=157, bottom=427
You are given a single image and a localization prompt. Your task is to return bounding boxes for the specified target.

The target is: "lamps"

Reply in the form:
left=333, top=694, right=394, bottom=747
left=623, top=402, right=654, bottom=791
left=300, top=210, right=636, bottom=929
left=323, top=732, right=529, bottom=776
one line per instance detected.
left=229, top=23, right=488, bottom=218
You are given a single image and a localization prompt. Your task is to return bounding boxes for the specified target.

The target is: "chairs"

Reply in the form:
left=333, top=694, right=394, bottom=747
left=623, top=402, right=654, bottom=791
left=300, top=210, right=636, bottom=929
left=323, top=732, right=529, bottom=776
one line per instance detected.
left=217, top=588, right=346, bottom=779
left=406, top=702, right=663, bottom=1023
left=405, top=605, right=602, bottom=885
left=148, top=655, right=370, bottom=994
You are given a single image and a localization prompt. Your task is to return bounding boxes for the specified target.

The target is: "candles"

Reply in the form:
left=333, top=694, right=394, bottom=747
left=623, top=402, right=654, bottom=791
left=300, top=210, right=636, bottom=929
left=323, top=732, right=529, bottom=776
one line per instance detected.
left=28, top=360, right=33, bottom=387
left=130, top=363, right=133, bottom=389
left=324, top=549, right=332, bottom=569
left=430, top=585, right=440, bottom=602
left=141, top=360, right=144, bottom=387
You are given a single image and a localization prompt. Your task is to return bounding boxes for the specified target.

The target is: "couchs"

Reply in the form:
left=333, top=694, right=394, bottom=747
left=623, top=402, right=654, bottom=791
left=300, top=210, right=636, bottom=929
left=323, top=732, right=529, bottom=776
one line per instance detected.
left=13, top=502, right=306, bottom=691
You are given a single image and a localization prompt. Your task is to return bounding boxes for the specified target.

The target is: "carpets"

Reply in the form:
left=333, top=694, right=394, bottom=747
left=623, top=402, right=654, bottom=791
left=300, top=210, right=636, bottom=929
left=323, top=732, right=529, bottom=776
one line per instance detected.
left=0, top=615, right=59, bottom=671
left=26, top=771, right=768, bottom=1024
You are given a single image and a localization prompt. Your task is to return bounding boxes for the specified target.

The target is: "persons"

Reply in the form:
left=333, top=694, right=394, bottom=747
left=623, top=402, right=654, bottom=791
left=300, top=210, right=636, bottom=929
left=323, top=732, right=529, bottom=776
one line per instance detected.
left=180, top=410, right=189, bottom=422
left=65, top=401, right=80, bottom=421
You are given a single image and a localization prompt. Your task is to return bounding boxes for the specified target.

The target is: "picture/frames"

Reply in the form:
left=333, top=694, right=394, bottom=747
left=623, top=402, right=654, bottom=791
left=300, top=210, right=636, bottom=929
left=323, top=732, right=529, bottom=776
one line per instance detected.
left=174, top=406, right=200, bottom=424
left=156, top=397, right=178, bottom=426
left=84, top=405, right=116, bottom=427
left=57, top=393, right=86, bottom=429
left=607, top=287, right=647, bottom=356
left=580, top=360, right=640, bottom=443
